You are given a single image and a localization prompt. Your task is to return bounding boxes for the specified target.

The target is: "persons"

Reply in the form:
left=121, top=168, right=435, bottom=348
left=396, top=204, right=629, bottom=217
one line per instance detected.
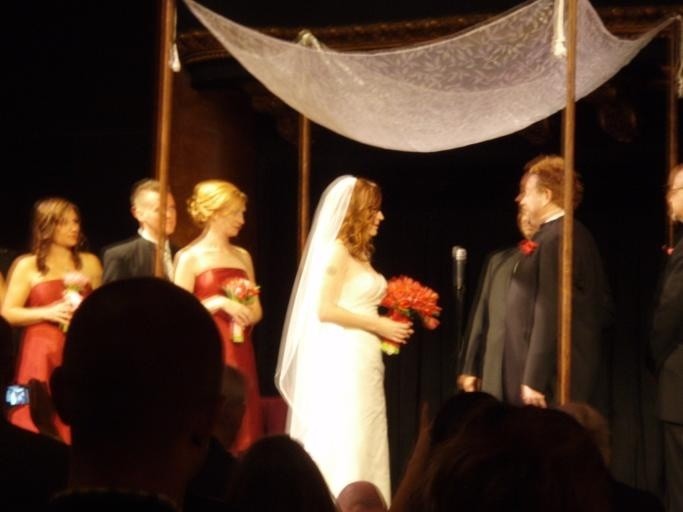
left=103, top=179, right=184, bottom=285
left=275, top=175, right=414, bottom=510
left=458, top=211, right=538, bottom=405
left=515, top=156, right=614, bottom=468
left=173, top=180, right=264, bottom=455
left=653, top=165, right=683, bottom=512
left=0, top=198, right=104, bottom=446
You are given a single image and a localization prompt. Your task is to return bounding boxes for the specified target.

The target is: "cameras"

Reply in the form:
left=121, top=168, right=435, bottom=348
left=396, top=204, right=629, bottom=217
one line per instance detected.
left=5, top=384, right=31, bottom=406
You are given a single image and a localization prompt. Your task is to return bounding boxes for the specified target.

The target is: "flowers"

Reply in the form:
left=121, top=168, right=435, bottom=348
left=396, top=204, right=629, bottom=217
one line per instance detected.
left=59, top=272, right=87, bottom=331
left=378, top=275, right=441, bottom=355
left=222, top=276, right=261, bottom=342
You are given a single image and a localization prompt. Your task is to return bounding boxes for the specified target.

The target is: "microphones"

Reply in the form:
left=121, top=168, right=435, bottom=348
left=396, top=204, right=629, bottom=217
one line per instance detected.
left=456, top=248, right=467, bottom=289
